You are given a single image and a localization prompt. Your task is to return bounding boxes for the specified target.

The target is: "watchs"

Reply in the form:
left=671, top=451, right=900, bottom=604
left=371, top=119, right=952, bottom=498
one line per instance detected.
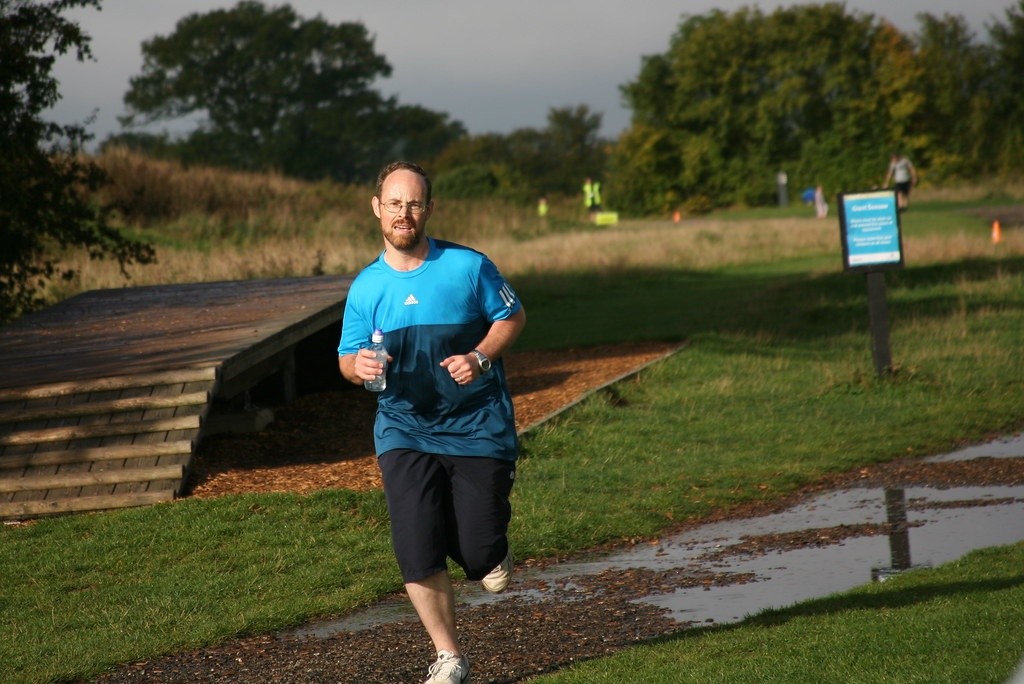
left=470, top=349, right=491, bottom=375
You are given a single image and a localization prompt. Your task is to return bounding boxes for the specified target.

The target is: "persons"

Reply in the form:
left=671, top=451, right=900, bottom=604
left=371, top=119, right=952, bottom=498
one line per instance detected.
left=582, top=176, right=605, bottom=223
left=815, top=184, right=828, bottom=223
left=537, top=197, right=550, bottom=231
left=776, top=171, right=789, bottom=211
left=338, top=160, right=527, bottom=684
left=882, top=152, right=918, bottom=212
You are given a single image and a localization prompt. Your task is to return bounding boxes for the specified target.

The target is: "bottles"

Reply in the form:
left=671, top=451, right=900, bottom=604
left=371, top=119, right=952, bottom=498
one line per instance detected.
left=364, top=329, right=387, bottom=393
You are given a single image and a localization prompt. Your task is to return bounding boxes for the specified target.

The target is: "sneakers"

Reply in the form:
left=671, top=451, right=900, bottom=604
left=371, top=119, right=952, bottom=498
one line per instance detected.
left=423, top=654, right=469, bottom=684
left=480, top=542, right=515, bottom=593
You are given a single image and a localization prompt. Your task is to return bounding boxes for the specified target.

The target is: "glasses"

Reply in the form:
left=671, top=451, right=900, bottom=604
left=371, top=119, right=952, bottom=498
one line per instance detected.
left=379, top=200, right=427, bottom=213
